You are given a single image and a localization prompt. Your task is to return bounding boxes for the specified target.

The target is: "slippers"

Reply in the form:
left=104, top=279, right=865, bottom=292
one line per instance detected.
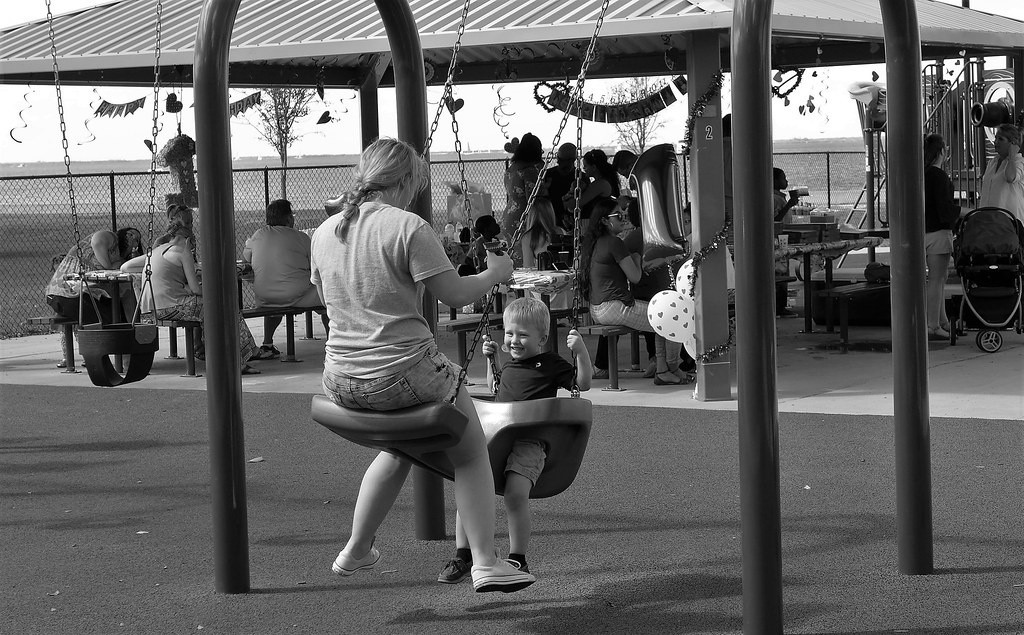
left=193, top=344, right=206, bottom=360
left=247, top=349, right=274, bottom=361
left=241, top=364, right=260, bottom=375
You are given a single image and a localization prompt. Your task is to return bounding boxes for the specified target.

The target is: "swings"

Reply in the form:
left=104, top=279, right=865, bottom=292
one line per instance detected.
left=310, top=0, right=610, bottom=499
left=46, top=0, right=162, bottom=388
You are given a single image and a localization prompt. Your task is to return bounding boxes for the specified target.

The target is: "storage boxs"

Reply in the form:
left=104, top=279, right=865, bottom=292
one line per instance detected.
left=810, top=267, right=867, bottom=325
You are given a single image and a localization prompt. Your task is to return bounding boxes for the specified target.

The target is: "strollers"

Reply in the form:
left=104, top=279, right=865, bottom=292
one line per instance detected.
left=947, top=205, right=1024, bottom=353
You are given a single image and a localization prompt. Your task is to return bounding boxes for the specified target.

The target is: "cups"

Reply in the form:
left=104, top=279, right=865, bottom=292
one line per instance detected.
left=778, top=235, right=788, bottom=248
left=789, top=190, right=799, bottom=198
left=454, top=232, right=460, bottom=242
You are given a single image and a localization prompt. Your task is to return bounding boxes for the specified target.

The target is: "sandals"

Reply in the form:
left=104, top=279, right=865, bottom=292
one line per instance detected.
left=653, top=371, right=689, bottom=385
left=672, top=368, right=694, bottom=382
left=941, top=322, right=967, bottom=336
left=928, top=326, right=958, bottom=339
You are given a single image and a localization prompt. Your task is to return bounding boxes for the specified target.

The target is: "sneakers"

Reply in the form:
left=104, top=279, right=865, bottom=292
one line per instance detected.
left=505, top=558, right=530, bottom=576
left=332, top=543, right=381, bottom=577
left=438, top=557, right=472, bottom=584
left=470, top=547, right=535, bottom=594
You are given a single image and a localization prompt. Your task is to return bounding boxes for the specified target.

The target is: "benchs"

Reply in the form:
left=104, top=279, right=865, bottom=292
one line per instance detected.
left=771, top=277, right=891, bottom=348
left=576, top=318, right=648, bottom=391
left=29, top=308, right=80, bottom=371
left=436, top=309, right=504, bottom=371
left=165, top=304, right=318, bottom=377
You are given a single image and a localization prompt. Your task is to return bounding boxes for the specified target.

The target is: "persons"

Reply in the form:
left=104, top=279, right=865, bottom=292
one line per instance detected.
left=922, top=132, right=977, bottom=341
left=465, top=215, right=500, bottom=274
left=437, top=296, right=593, bottom=584
left=121, top=204, right=274, bottom=375
left=580, top=197, right=734, bottom=386
left=500, top=131, right=638, bottom=329
left=774, top=167, right=800, bottom=319
left=45, top=226, right=143, bottom=325
left=980, top=124, right=1024, bottom=228
left=243, top=200, right=329, bottom=358
left=311, top=139, right=536, bottom=593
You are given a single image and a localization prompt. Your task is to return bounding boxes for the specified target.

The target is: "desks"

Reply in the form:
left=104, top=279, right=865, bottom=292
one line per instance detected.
left=727, top=235, right=884, bottom=330
left=502, top=266, right=584, bottom=355
left=65, top=260, right=252, bottom=376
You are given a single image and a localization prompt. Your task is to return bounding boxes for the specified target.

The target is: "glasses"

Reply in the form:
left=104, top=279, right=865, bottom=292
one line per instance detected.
left=557, top=155, right=574, bottom=162
left=290, top=212, right=297, bottom=216
left=607, top=212, right=623, bottom=220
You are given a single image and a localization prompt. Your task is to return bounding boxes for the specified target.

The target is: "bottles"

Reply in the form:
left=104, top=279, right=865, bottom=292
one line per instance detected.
left=444, top=221, right=454, bottom=244
left=791, top=202, right=815, bottom=215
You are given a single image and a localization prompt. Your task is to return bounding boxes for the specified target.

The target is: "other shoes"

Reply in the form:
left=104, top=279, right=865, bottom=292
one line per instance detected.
left=261, top=345, right=280, bottom=358
left=644, top=356, right=657, bottom=378
left=779, top=307, right=798, bottom=317
left=775, top=313, right=780, bottom=319
left=592, top=363, right=609, bottom=379
